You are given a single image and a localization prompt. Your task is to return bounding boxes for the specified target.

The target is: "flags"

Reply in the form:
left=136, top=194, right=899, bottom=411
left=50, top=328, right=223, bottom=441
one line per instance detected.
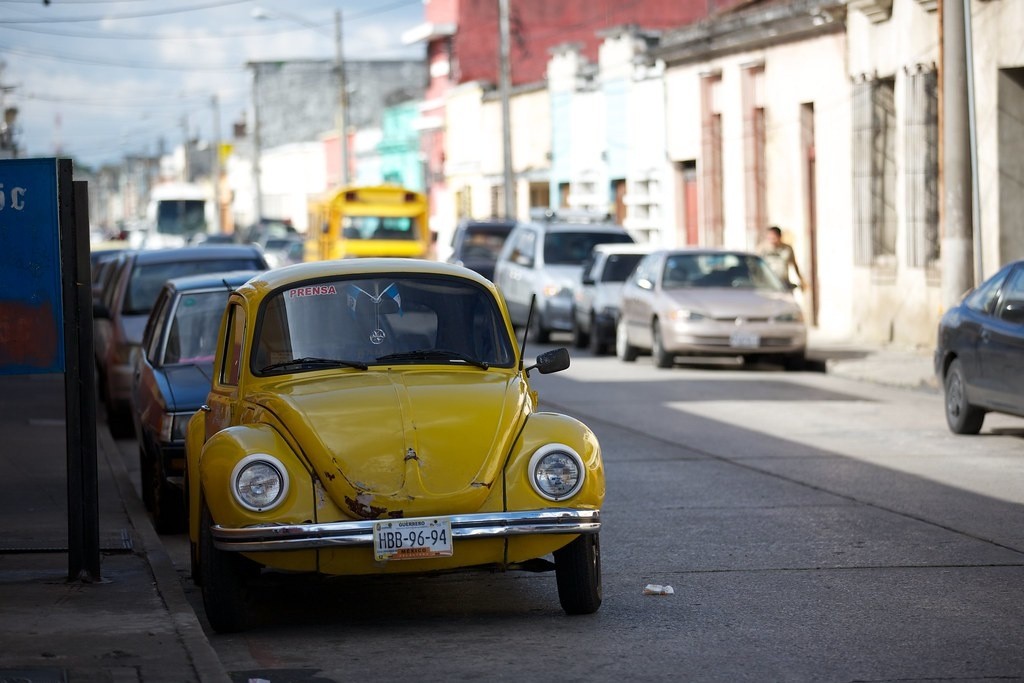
left=385, top=284, right=403, bottom=317
left=346, top=286, right=360, bottom=320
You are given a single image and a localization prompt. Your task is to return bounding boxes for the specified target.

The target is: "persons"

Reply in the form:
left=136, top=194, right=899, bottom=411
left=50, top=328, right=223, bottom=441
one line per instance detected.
left=757, top=226, right=806, bottom=301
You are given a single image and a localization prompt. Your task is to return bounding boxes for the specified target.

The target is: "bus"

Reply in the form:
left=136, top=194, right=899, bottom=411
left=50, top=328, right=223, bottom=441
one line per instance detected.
left=304, top=181, right=436, bottom=266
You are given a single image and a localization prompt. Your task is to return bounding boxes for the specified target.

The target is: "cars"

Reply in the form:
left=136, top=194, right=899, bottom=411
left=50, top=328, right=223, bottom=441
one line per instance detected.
left=185, top=256, right=606, bottom=634
left=933, top=260, right=1024, bottom=434
left=616, top=250, right=807, bottom=371
left=133, top=268, right=261, bottom=538
left=441, top=216, right=513, bottom=281
left=569, top=239, right=657, bottom=356
left=92, top=243, right=269, bottom=443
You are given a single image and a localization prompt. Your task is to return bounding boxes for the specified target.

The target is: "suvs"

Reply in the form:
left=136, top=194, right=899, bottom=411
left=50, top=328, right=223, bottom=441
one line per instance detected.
left=495, top=205, right=634, bottom=342
